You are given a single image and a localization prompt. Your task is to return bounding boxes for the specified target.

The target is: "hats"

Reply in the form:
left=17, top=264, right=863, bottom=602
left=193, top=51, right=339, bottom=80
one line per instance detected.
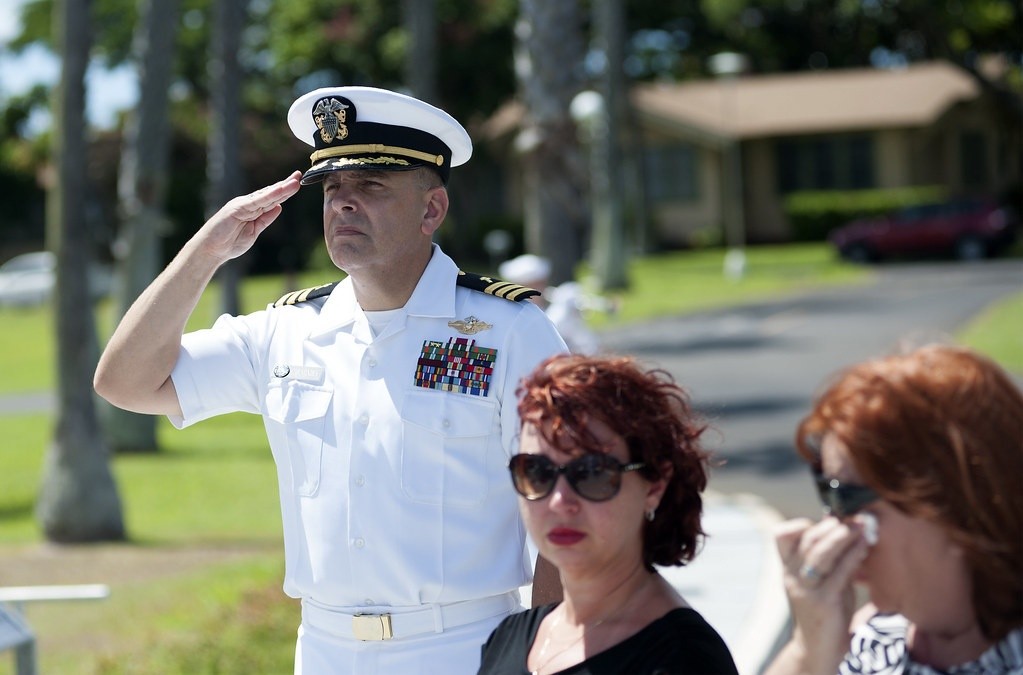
left=285, top=84, right=473, bottom=186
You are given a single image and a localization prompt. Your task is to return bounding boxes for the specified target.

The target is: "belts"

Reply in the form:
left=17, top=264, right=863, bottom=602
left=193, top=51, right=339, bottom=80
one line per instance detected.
left=301, top=588, right=522, bottom=642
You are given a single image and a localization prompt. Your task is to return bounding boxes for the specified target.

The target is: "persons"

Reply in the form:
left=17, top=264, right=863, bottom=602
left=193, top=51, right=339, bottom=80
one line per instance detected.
left=470, top=350, right=742, bottom=675
left=93, top=83, right=573, bottom=675
left=754, top=344, right=1023, bottom=675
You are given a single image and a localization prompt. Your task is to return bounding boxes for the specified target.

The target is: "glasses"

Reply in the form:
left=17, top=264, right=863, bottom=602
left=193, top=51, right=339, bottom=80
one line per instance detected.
left=807, top=470, right=887, bottom=519
left=508, top=452, right=646, bottom=503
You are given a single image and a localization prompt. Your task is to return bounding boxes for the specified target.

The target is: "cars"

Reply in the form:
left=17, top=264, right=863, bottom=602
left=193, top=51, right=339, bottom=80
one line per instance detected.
left=832, top=197, right=1022, bottom=267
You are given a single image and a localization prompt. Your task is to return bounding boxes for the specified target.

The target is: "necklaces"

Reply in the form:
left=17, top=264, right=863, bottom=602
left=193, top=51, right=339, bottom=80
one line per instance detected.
left=915, top=617, right=982, bottom=643
left=528, top=568, right=658, bottom=675
